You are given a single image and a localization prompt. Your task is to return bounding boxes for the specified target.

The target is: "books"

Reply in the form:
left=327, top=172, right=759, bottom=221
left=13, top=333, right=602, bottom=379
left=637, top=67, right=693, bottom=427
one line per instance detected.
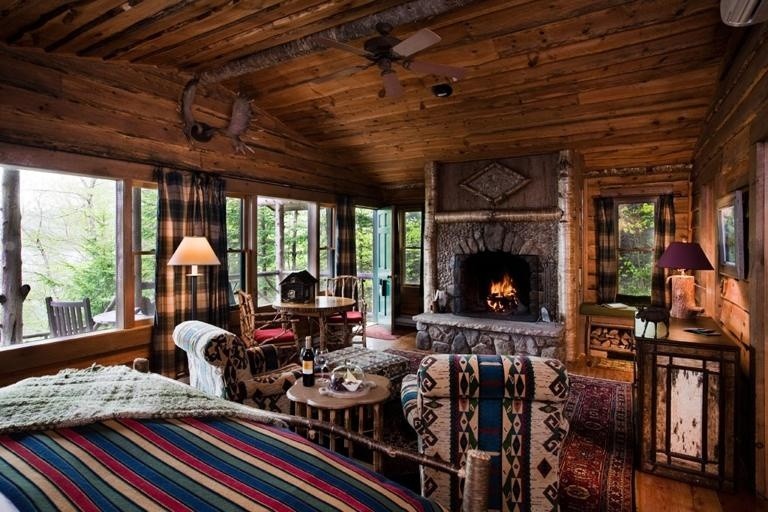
left=595, top=302, right=628, bottom=310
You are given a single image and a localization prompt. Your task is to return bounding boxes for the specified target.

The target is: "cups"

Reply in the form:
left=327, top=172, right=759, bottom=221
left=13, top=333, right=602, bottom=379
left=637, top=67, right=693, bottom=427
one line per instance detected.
left=299, top=346, right=313, bottom=364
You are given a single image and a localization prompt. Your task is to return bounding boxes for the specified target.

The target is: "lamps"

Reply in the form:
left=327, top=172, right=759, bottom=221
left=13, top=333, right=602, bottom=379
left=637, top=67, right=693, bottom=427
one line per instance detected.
left=654, top=242, right=715, bottom=322
left=165, top=235, right=222, bottom=321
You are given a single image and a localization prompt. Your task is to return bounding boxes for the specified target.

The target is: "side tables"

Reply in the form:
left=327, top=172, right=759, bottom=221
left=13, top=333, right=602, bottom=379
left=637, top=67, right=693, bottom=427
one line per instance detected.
left=286, top=372, right=392, bottom=473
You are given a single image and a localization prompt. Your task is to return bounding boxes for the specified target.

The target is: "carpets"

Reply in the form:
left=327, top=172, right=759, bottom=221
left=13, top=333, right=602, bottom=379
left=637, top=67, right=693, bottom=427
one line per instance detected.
left=381, top=346, right=636, bottom=511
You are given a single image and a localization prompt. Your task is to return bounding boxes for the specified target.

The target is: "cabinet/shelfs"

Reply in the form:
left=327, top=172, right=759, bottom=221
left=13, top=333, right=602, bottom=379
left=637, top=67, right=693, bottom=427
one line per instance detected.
left=633, top=311, right=740, bottom=493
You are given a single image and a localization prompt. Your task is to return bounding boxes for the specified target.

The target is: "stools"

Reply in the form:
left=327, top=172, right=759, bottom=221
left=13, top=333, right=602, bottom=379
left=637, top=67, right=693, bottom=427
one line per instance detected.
left=315, top=345, right=411, bottom=385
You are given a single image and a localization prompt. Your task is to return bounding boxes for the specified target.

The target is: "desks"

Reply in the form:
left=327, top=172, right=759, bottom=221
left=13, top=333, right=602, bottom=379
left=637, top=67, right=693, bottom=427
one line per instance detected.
left=91, top=308, right=155, bottom=330
left=272, top=296, right=357, bottom=352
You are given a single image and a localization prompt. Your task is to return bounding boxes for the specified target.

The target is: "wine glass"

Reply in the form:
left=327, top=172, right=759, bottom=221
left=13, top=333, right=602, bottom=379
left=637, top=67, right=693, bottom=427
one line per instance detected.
left=314, top=347, right=330, bottom=385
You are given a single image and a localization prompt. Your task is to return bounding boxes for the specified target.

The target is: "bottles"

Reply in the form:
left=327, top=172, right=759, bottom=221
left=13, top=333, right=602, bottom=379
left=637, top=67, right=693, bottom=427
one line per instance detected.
left=300, top=335, right=315, bottom=387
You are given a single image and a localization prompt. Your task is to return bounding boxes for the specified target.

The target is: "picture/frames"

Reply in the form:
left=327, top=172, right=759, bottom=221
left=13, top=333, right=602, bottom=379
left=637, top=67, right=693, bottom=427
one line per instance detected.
left=714, top=189, right=749, bottom=283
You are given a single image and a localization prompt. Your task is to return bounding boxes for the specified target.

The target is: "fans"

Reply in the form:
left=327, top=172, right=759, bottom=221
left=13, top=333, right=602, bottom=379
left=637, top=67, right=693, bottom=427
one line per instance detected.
left=317, top=23, right=472, bottom=97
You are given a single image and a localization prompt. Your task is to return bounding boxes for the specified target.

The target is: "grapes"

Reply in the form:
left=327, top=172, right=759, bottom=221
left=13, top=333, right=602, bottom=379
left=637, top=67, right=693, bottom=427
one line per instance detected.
left=330, top=377, right=345, bottom=391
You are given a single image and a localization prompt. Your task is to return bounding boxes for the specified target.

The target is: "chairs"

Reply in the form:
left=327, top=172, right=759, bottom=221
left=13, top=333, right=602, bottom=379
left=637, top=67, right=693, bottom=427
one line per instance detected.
left=400, top=352, right=569, bottom=512
left=323, top=274, right=367, bottom=349
left=45, top=295, right=94, bottom=338
left=171, top=320, right=303, bottom=415
left=233, top=289, right=299, bottom=349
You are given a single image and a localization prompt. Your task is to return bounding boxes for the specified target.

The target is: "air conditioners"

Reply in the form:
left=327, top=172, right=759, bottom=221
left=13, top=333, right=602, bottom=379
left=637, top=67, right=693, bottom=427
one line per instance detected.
left=720, top=0, right=768, bottom=28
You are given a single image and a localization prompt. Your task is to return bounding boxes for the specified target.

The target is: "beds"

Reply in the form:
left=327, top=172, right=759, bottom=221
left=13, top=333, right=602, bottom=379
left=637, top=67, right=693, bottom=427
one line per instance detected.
left=1, top=357, right=493, bottom=512
